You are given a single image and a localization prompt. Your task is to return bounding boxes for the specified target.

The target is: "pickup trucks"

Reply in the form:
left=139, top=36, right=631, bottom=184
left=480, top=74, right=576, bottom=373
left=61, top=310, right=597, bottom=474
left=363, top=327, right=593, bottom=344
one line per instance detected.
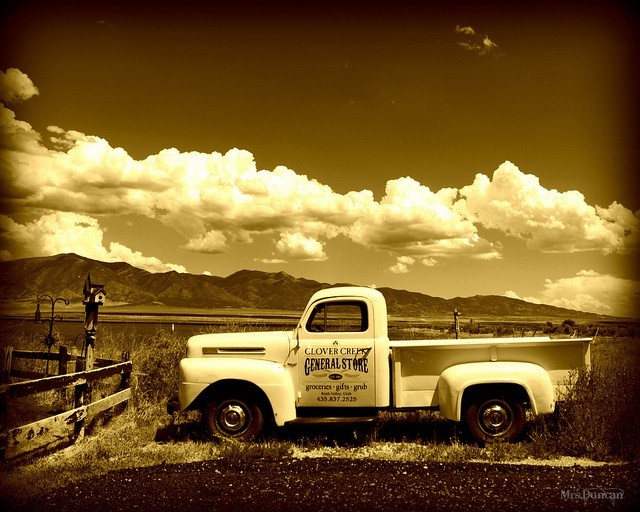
left=166, top=285, right=596, bottom=446
